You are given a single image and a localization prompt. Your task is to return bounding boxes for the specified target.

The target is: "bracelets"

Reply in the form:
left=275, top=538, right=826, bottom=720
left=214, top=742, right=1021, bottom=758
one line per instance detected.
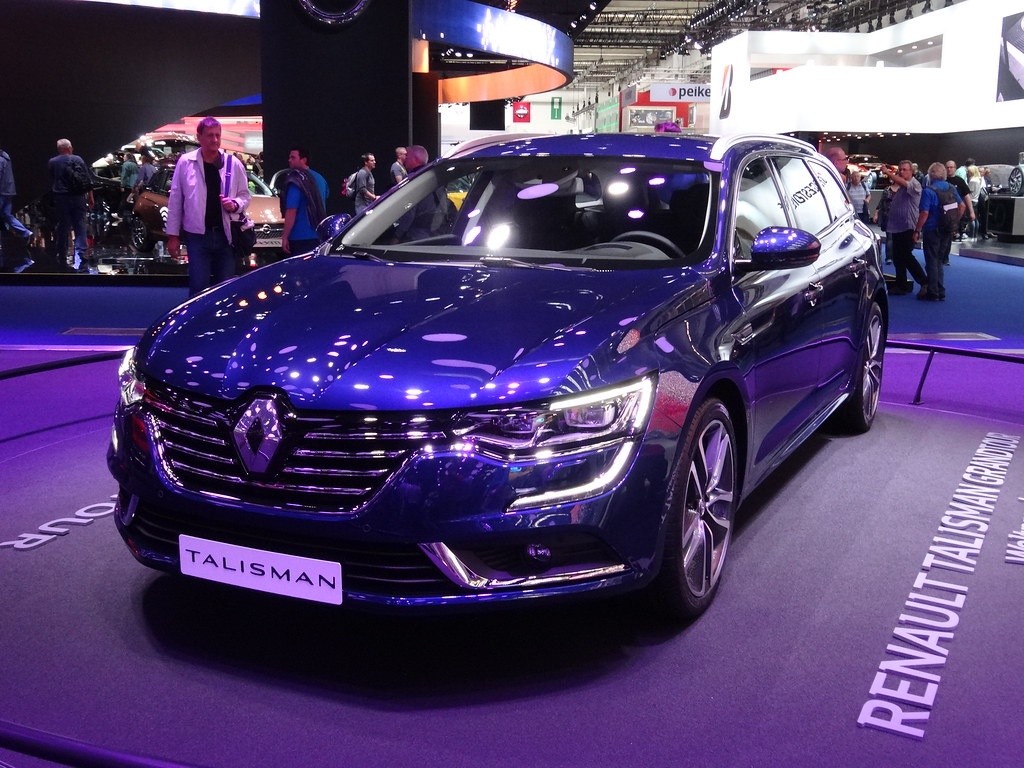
left=885, top=169, right=891, bottom=174
left=916, top=229, right=921, bottom=232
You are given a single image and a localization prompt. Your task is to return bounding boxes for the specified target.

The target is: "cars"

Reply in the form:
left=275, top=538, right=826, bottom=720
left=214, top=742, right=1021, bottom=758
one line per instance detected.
left=978, top=151, right=1024, bottom=196
left=129, top=166, right=290, bottom=266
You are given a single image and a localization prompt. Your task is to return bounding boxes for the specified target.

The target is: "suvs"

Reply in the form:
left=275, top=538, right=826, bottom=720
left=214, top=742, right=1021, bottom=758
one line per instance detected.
left=105, top=131, right=886, bottom=623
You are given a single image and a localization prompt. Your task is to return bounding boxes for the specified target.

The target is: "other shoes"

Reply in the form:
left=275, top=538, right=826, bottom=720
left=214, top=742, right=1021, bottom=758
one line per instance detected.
left=987, top=232, right=998, bottom=238
left=982, top=234, right=989, bottom=240
left=57, top=254, right=67, bottom=266
left=24, top=232, right=34, bottom=245
left=916, top=284, right=928, bottom=295
left=925, top=294, right=946, bottom=302
left=962, top=233, right=968, bottom=239
left=73, top=255, right=88, bottom=263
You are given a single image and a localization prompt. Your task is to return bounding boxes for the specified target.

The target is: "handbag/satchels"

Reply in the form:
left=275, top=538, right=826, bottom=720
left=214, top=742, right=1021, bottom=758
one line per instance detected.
left=230, top=216, right=257, bottom=258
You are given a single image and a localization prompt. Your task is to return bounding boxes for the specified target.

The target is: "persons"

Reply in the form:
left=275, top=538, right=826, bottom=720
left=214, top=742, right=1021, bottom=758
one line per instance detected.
left=169, top=116, right=252, bottom=296
left=873, top=158, right=997, bottom=266
left=0, top=150, right=34, bottom=236
left=405, top=146, right=430, bottom=174
left=826, top=145, right=849, bottom=173
left=846, top=173, right=872, bottom=222
left=132, top=156, right=157, bottom=192
left=48, top=139, right=95, bottom=270
left=281, top=148, right=329, bottom=255
left=914, top=162, right=965, bottom=301
left=880, top=159, right=927, bottom=299
left=121, top=154, right=138, bottom=198
left=353, top=151, right=381, bottom=216
left=390, top=146, right=407, bottom=185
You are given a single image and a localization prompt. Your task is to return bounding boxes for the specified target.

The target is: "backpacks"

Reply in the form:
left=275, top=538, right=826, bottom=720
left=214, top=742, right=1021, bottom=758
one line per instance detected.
left=342, top=171, right=371, bottom=199
left=929, top=185, right=959, bottom=233
